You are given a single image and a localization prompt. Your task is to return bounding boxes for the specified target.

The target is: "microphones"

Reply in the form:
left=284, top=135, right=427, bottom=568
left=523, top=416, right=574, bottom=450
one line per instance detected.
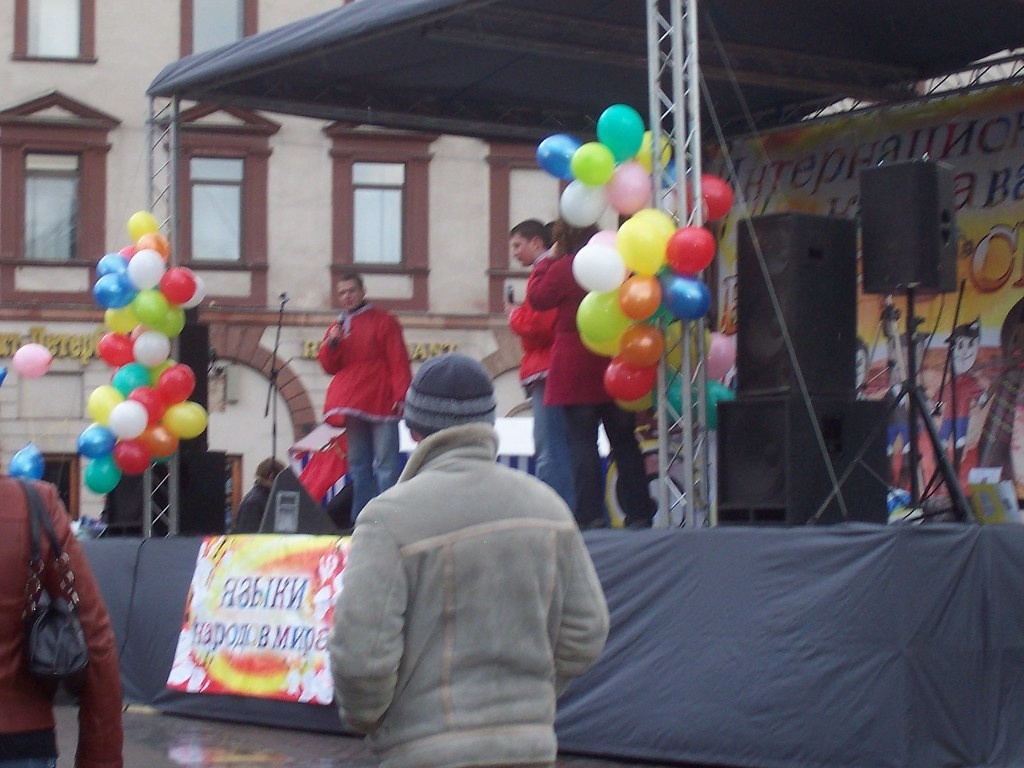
left=507, top=284, right=514, bottom=307
left=329, top=315, right=346, bottom=349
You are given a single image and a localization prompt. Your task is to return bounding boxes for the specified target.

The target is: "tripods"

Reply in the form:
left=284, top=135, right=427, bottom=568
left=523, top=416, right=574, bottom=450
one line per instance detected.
left=807, top=290, right=978, bottom=526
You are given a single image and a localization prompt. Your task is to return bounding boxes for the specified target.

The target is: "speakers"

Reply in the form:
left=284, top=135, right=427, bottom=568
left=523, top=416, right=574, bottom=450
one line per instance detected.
left=860, top=159, right=957, bottom=294
left=103, top=449, right=227, bottom=538
left=736, top=212, right=858, bottom=399
left=164, top=321, right=208, bottom=451
left=259, top=466, right=342, bottom=536
left=716, top=398, right=889, bottom=529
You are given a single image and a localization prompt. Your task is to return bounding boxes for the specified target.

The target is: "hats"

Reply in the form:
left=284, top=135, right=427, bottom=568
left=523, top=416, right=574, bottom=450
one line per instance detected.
left=257, top=458, right=284, bottom=473
left=403, top=352, right=496, bottom=434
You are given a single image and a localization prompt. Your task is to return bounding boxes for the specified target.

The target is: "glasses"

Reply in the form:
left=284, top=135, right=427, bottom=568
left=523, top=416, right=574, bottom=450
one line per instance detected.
left=337, top=287, right=356, bottom=295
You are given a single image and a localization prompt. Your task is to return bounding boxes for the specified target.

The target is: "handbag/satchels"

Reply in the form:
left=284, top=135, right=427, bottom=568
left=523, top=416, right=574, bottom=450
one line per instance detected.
left=17, top=476, right=89, bottom=706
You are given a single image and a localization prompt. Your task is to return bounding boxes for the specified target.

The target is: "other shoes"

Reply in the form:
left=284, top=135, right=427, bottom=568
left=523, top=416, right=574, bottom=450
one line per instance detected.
left=623, top=518, right=652, bottom=530
left=578, top=518, right=606, bottom=528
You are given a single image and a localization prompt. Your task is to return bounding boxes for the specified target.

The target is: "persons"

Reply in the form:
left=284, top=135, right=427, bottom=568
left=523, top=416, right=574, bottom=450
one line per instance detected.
left=0, top=474, right=135, bottom=768
left=502, top=216, right=658, bottom=530
left=326, top=351, right=610, bottom=768
left=317, top=271, right=412, bottom=535
left=232, top=456, right=287, bottom=533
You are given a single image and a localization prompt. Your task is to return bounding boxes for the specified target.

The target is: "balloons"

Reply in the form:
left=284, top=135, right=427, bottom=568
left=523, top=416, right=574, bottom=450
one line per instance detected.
left=11, top=341, right=55, bottom=378
left=76, top=211, right=207, bottom=494
left=7, top=441, right=46, bottom=481
left=536, top=103, right=736, bottom=430
